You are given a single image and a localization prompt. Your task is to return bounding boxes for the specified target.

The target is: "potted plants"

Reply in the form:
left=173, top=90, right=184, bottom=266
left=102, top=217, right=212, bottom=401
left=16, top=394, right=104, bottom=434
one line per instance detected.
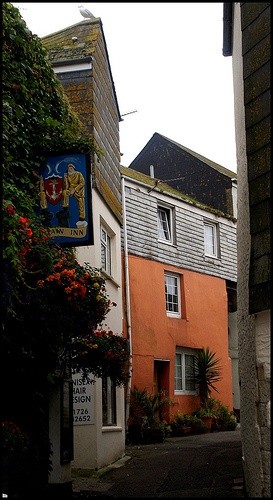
left=134, top=388, right=169, bottom=443
left=174, top=411, right=192, bottom=437
left=196, top=409, right=212, bottom=434
